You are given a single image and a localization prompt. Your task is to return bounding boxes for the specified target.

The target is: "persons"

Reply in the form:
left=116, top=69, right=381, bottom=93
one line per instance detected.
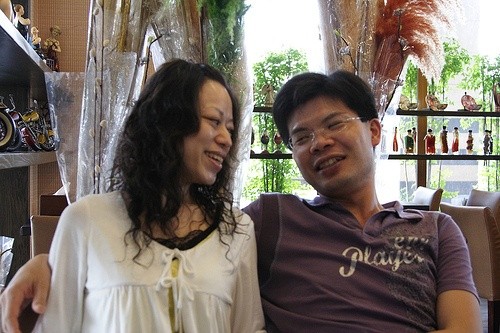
left=30, top=57, right=271, bottom=333
left=390, top=125, right=495, bottom=156
left=0, top=69, right=488, bottom=333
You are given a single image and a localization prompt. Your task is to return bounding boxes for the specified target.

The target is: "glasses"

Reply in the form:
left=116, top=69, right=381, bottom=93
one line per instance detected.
left=287, top=115, right=371, bottom=150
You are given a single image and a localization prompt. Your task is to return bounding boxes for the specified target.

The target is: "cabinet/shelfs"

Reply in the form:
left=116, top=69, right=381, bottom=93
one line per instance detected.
left=250, top=107, right=500, bottom=159
left=0, top=7, right=75, bottom=169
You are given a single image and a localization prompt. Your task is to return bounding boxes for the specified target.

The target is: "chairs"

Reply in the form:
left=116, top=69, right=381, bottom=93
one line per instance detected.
left=19, top=185, right=500, bottom=333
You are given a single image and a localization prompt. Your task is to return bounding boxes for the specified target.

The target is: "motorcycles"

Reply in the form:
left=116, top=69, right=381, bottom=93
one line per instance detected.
left=0, top=94, right=60, bottom=153
